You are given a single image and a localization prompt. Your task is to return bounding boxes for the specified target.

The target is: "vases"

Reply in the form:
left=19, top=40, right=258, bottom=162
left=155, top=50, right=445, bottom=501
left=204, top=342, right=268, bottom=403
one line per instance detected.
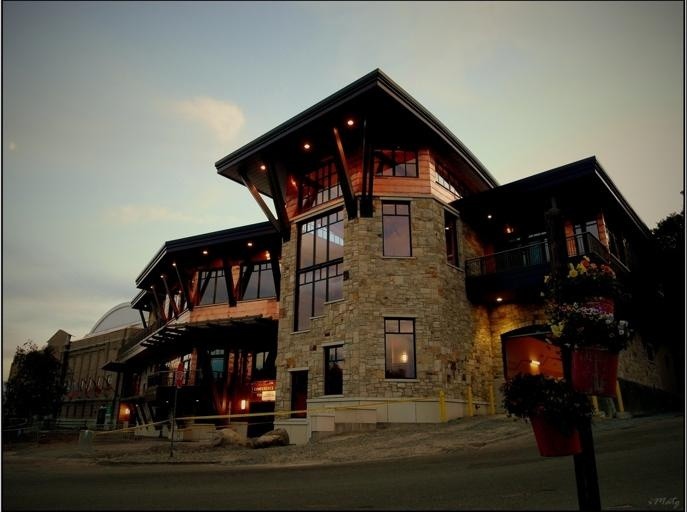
left=586, top=296, right=614, bottom=316
left=529, top=418, right=584, bottom=456
left=571, top=347, right=618, bottom=400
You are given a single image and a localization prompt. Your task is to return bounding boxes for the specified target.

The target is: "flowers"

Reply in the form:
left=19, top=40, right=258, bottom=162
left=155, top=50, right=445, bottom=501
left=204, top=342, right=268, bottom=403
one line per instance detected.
left=545, top=253, right=617, bottom=304
left=540, top=290, right=634, bottom=344
left=498, top=371, right=595, bottom=425
